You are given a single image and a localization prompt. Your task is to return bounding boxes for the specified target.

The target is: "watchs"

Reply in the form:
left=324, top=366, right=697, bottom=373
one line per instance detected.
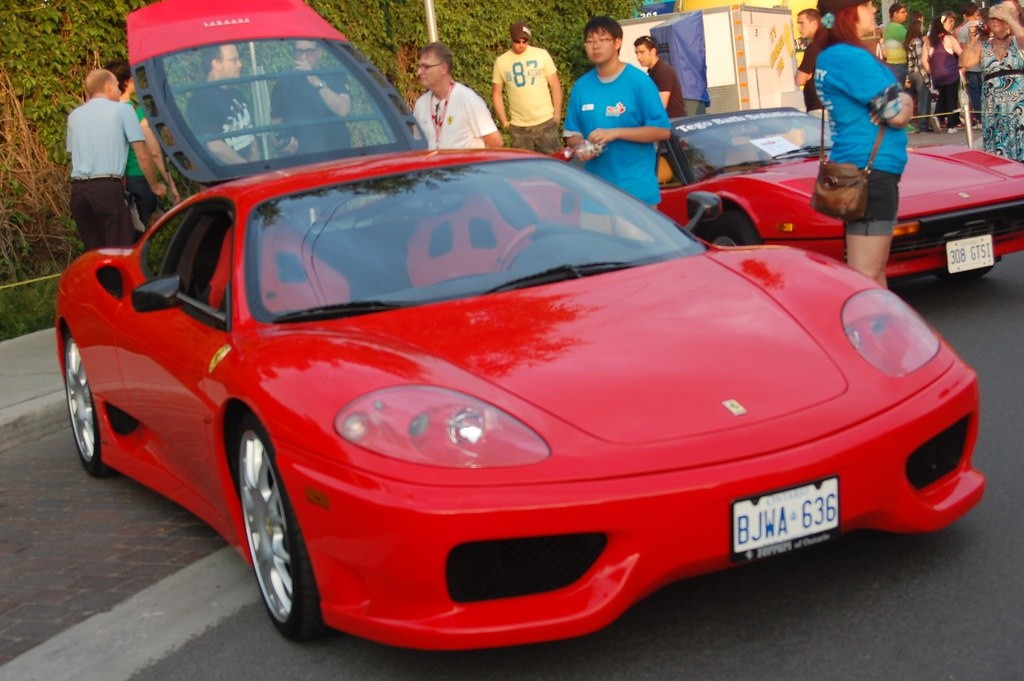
left=315, top=80, right=327, bottom=90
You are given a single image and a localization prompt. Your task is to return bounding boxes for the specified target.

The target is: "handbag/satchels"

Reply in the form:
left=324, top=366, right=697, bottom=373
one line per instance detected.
left=124, top=190, right=145, bottom=235
left=809, top=158, right=868, bottom=220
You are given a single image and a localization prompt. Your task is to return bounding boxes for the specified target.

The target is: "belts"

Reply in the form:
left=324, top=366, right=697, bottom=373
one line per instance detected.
left=72, top=177, right=120, bottom=183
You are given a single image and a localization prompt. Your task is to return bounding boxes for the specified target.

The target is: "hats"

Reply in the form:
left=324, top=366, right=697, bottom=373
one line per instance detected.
left=510, top=22, right=530, bottom=40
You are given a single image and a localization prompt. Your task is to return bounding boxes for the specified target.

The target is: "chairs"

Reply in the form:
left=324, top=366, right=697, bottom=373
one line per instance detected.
left=403, top=184, right=523, bottom=290
left=194, top=214, right=352, bottom=314
left=721, top=134, right=758, bottom=167
left=657, top=155, right=679, bottom=190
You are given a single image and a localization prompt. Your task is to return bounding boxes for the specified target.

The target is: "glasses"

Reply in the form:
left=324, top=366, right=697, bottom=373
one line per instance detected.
left=639, top=36, right=652, bottom=42
left=897, top=11, right=906, bottom=14
left=216, top=56, right=242, bottom=63
left=434, top=103, right=440, bottom=124
left=583, top=38, right=614, bottom=47
left=514, top=39, right=527, bottom=43
left=415, top=63, right=443, bottom=71
left=292, top=48, right=317, bottom=58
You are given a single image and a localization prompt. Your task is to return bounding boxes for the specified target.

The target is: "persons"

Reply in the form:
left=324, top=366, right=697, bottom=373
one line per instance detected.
left=561, top=17, right=671, bottom=244
left=65, top=57, right=180, bottom=250
left=812, top=0, right=914, bottom=290
left=410, top=41, right=505, bottom=150
left=881, top=2, right=985, bottom=134
left=268, top=39, right=354, bottom=158
left=957, top=0, right=1024, bottom=162
left=185, top=43, right=263, bottom=166
left=633, top=36, right=688, bottom=118
left=795, top=9, right=829, bottom=123
left=490, top=22, right=563, bottom=155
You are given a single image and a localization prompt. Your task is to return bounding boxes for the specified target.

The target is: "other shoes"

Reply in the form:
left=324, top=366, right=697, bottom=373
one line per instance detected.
left=973, top=118, right=983, bottom=130
left=931, top=116, right=942, bottom=132
left=905, top=122, right=918, bottom=133
left=947, top=128, right=957, bottom=134
left=940, top=121, right=949, bottom=129
left=920, top=126, right=931, bottom=136
left=954, top=123, right=966, bottom=129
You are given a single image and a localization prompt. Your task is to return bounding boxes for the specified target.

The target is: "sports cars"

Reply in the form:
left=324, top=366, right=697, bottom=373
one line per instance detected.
left=549, top=107, right=1024, bottom=282
left=55, top=1, right=985, bottom=647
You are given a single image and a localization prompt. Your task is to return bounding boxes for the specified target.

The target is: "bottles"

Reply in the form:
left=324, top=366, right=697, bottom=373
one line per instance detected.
left=563, top=140, right=609, bottom=159
